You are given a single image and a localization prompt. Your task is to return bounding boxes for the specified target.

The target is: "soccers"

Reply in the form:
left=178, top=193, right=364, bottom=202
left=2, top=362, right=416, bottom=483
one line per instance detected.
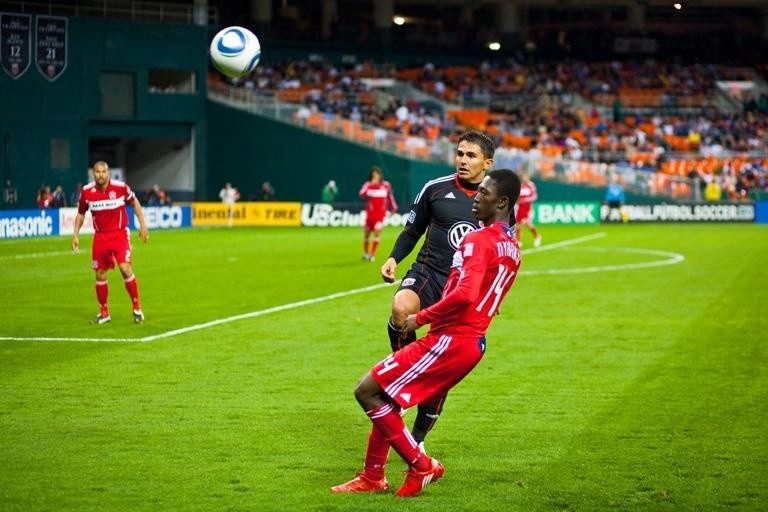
left=210, top=25, right=260, bottom=78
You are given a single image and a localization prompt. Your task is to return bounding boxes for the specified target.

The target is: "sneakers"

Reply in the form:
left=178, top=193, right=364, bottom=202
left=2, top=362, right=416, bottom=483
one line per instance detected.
left=330, top=473, right=390, bottom=492
left=133, top=309, right=144, bottom=323
left=394, top=458, right=445, bottom=497
left=90, top=313, right=111, bottom=324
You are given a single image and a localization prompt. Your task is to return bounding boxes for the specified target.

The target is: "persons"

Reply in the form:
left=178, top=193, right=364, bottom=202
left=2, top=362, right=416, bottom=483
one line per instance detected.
left=515, top=168, right=542, bottom=247
left=35, top=183, right=54, bottom=208
left=602, top=177, right=626, bottom=222
left=358, top=167, right=397, bottom=260
left=143, top=184, right=165, bottom=207
left=209, top=62, right=767, bottom=203
left=328, top=169, right=523, bottom=498
left=71, top=183, right=83, bottom=207
left=51, top=184, right=67, bottom=208
left=164, top=194, right=174, bottom=206
left=70, top=161, right=147, bottom=325
left=380, top=132, right=514, bottom=455
